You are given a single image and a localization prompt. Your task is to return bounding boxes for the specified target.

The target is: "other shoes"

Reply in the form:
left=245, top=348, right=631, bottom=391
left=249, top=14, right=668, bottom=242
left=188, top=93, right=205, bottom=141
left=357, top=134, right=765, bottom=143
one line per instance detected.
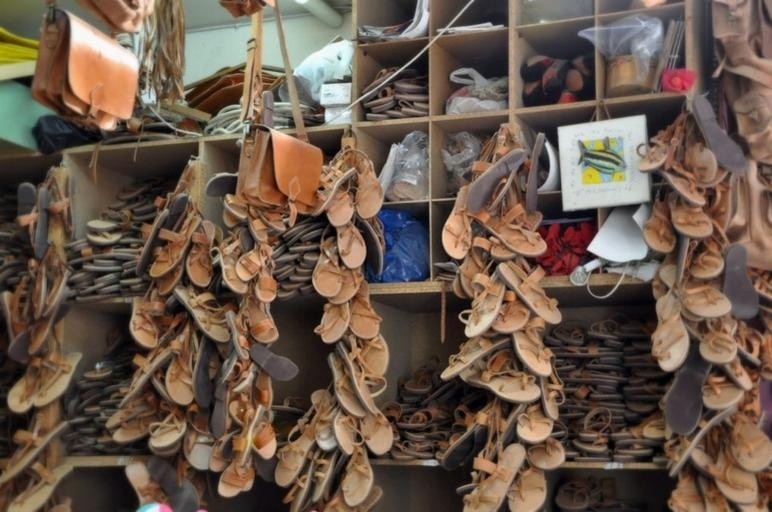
left=522, top=54, right=593, bottom=108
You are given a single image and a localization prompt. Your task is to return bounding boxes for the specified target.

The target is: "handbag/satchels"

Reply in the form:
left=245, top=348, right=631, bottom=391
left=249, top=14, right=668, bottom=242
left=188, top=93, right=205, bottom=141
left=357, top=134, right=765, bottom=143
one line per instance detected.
left=445, top=67, right=509, bottom=114
left=236, top=9, right=323, bottom=228
left=30, top=1, right=140, bottom=132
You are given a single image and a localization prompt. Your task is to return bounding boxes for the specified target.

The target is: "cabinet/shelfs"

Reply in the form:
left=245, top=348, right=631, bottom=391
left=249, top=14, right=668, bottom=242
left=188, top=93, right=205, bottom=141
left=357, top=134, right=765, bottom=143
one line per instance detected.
left=352, top=1, right=691, bottom=473
left=0, top=124, right=350, bottom=467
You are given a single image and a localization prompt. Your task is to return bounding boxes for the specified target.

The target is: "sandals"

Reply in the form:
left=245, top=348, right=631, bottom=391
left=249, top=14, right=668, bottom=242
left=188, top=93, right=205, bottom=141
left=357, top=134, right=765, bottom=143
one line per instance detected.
left=368, top=98, right=765, bottom=512
left=2, top=128, right=394, bottom=512
left=362, top=64, right=428, bottom=121
